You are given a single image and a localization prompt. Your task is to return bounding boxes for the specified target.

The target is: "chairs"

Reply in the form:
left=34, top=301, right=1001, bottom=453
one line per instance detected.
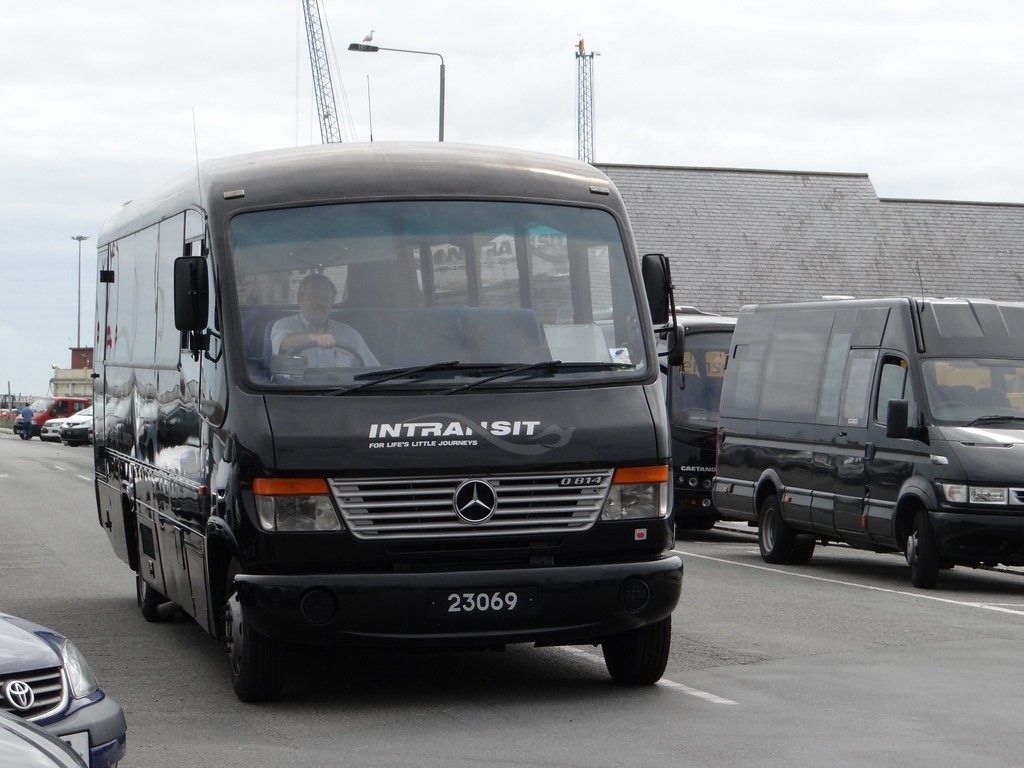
left=237, top=302, right=549, bottom=369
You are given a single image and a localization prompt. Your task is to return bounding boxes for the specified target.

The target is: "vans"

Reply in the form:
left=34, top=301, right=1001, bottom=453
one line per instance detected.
left=58, top=405, right=94, bottom=447
left=710, top=297, right=1024, bottom=588
left=13, top=397, right=91, bottom=439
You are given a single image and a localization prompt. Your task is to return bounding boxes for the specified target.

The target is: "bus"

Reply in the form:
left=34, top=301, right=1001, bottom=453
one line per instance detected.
left=557, top=305, right=738, bottom=530
left=94, top=141, right=683, bottom=703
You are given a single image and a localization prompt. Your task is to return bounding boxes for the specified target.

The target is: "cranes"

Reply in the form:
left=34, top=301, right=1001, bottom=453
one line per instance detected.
left=574, top=33, right=601, bottom=163
left=302, top=0, right=342, bottom=144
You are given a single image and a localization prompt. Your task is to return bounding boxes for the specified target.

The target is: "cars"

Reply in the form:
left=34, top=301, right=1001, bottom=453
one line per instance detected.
left=0, top=612, right=126, bottom=767
left=40, top=418, right=67, bottom=442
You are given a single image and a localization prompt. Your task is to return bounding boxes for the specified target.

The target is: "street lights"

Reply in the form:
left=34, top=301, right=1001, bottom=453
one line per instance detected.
left=348, top=43, right=446, bottom=141
left=73, top=236, right=91, bottom=348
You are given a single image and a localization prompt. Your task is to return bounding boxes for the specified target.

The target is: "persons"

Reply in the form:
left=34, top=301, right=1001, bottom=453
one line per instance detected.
left=21, top=403, right=34, bottom=440
left=271, top=274, right=381, bottom=370
